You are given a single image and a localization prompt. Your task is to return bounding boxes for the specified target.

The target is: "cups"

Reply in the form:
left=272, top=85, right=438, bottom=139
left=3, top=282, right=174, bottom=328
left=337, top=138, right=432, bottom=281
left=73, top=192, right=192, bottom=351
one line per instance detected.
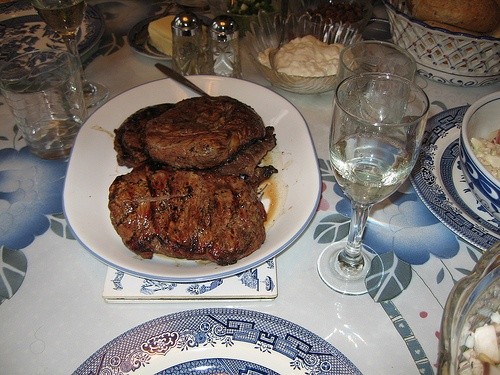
left=0, top=49, right=84, bottom=164
left=337, top=41, right=416, bottom=86
left=362, top=18, right=393, bottom=42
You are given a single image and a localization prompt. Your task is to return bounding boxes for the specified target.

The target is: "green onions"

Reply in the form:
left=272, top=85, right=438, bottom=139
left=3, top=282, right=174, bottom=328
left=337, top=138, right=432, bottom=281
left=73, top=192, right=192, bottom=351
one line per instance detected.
left=230, top=0, right=274, bottom=16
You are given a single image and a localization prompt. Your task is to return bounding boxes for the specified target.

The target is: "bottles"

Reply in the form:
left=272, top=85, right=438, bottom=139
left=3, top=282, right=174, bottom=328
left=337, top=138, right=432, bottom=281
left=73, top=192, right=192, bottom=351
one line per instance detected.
left=171, top=11, right=212, bottom=76
left=208, top=15, right=240, bottom=79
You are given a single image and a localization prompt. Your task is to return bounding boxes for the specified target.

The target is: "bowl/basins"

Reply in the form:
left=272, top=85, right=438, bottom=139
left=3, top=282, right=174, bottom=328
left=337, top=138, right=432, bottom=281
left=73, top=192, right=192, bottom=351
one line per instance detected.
left=243, top=9, right=364, bottom=94
left=282, top=1, right=371, bottom=34
left=199, top=0, right=285, bottom=40
left=459, top=91, right=500, bottom=223
left=437, top=238, right=500, bottom=375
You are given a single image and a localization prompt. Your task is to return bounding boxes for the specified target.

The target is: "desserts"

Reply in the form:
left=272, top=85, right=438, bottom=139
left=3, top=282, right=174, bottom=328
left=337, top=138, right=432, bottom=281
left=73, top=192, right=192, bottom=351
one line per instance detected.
left=147, top=14, right=207, bottom=56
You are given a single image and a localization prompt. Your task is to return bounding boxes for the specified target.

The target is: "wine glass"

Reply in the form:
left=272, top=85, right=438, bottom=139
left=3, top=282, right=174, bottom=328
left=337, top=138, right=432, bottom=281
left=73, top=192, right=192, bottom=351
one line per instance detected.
left=317, top=72, right=430, bottom=295
left=31, top=0, right=109, bottom=109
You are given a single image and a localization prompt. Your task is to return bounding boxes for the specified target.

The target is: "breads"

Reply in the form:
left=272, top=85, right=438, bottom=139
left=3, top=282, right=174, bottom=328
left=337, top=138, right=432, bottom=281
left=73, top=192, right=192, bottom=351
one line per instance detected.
left=402, top=0, right=500, bottom=41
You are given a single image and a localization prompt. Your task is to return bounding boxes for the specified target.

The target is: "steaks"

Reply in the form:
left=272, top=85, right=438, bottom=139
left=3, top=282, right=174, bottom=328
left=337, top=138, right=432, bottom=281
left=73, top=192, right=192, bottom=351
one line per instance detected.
left=106, top=96, right=269, bottom=265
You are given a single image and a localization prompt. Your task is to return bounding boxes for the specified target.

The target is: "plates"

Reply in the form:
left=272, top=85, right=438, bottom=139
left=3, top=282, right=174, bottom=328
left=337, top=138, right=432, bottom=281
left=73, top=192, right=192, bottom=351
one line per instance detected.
left=71, top=308, right=361, bottom=375
left=406, top=105, right=500, bottom=252
left=127, top=13, right=212, bottom=61
left=62, top=74, right=321, bottom=282
left=0, top=0, right=106, bottom=80
